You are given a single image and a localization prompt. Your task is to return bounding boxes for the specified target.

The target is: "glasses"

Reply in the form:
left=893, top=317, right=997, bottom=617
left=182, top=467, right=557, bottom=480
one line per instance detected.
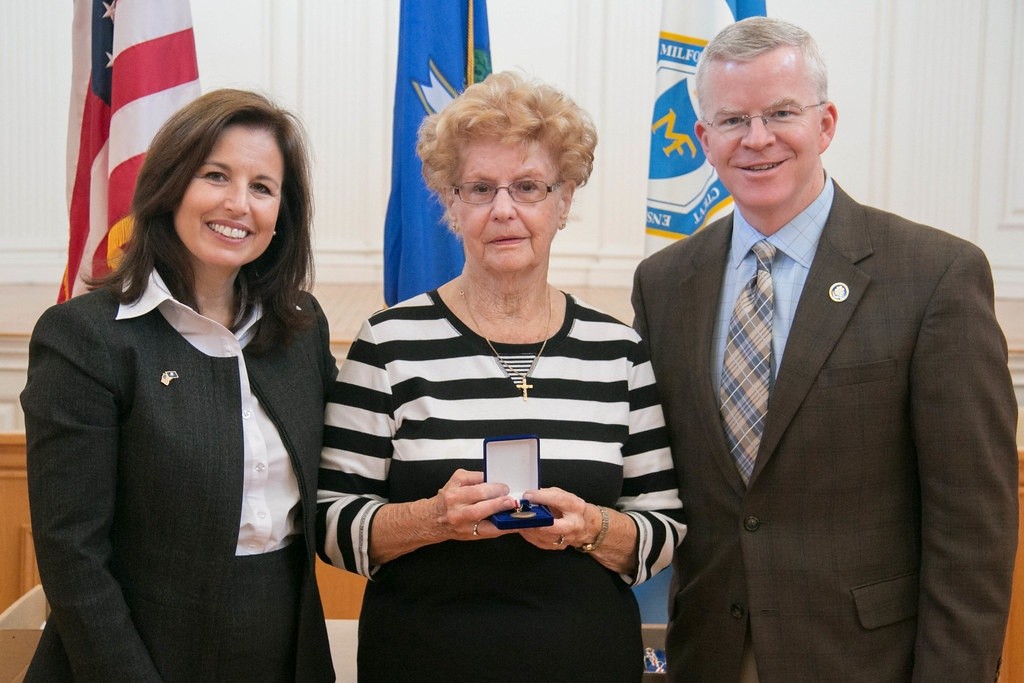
left=453, top=180, right=565, bottom=205
left=706, top=102, right=825, bottom=128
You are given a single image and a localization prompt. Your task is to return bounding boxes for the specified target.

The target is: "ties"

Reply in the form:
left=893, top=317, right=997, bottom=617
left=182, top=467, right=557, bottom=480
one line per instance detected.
left=720, top=237, right=780, bottom=489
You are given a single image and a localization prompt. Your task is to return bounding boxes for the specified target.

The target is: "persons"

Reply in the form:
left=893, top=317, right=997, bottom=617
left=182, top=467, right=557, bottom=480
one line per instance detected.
left=315, top=71, right=688, bottom=683
left=20, top=88, right=340, bottom=683
left=632, top=18, right=1019, bottom=683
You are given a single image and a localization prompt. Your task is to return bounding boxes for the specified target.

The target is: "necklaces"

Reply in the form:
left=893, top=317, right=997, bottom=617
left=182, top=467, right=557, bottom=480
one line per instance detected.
left=460, top=284, right=551, bottom=399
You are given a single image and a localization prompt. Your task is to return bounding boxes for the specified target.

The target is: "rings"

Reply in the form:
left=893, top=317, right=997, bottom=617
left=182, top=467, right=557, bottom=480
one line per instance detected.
left=555, top=533, right=565, bottom=546
left=472, top=520, right=478, bottom=537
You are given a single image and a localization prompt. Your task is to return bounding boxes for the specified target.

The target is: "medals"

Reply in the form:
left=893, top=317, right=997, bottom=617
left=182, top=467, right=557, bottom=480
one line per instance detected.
left=511, top=499, right=537, bottom=518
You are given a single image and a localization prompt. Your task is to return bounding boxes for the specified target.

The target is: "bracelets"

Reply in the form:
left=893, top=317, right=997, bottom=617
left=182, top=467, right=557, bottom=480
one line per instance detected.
left=581, top=505, right=608, bottom=551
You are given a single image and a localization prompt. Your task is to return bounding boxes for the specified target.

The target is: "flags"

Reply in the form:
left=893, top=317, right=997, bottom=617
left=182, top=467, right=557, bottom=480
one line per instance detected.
left=645, top=1, right=765, bottom=236
left=57, top=1, right=202, bottom=310
left=385, top=0, right=494, bottom=302
